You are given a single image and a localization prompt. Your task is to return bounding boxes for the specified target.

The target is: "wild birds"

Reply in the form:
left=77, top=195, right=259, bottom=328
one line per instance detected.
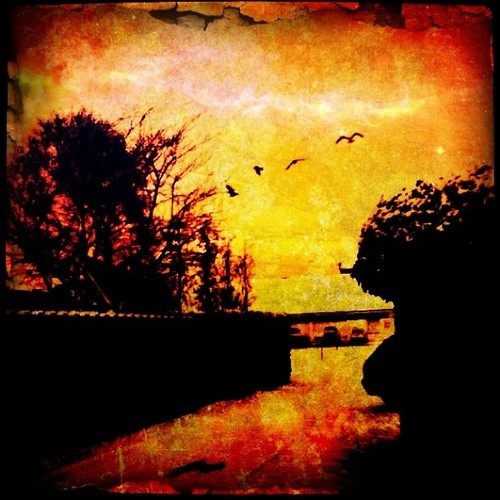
left=336, top=132, right=364, bottom=145
left=252, top=165, right=264, bottom=175
left=285, top=158, right=307, bottom=171
left=225, top=184, right=239, bottom=197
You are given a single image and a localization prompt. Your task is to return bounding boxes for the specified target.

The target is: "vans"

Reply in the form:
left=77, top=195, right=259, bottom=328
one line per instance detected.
left=322, top=327, right=341, bottom=347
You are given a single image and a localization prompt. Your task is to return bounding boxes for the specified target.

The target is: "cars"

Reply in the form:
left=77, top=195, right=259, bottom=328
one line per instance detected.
left=349, top=331, right=368, bottom=345
left=286, top=325, right=309, bottom=348
left=309, top=336, right=324, bottom=348
